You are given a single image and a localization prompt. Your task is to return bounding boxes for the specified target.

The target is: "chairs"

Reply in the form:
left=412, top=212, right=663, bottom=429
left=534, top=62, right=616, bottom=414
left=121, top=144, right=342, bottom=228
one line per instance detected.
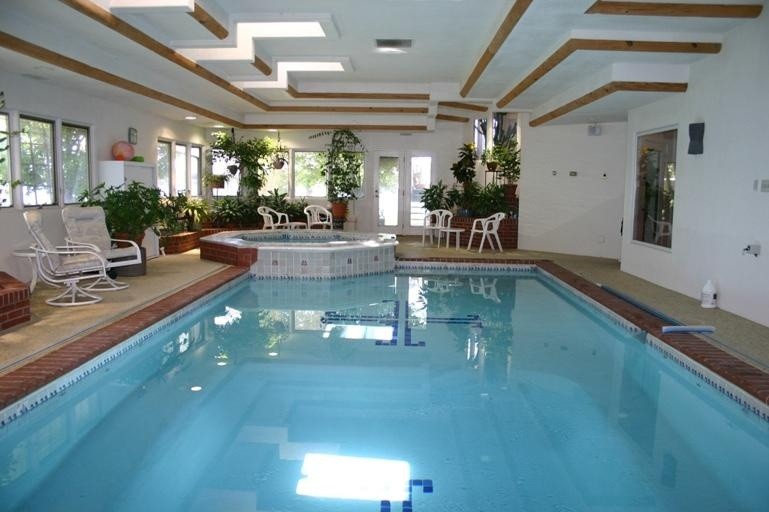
left=11, top=205, right=144, bottom=308
left=421, top=209, right=507, bottom=254
left=256, top=204, right=333, bottom=230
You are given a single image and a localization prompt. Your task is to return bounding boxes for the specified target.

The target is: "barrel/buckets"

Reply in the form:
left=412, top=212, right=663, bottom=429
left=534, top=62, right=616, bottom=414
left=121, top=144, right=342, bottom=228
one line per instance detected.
left=701, top=278, right=718, bottom=309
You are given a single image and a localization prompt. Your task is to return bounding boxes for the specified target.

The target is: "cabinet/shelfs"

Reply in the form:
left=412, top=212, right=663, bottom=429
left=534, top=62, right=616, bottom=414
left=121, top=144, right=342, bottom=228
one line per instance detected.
left=99, top=160, right=159, bottom=260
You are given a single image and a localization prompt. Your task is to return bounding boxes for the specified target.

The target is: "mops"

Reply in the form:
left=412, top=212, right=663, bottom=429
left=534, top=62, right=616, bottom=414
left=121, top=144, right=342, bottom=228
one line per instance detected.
left=595, top=282, right=716, bottom=334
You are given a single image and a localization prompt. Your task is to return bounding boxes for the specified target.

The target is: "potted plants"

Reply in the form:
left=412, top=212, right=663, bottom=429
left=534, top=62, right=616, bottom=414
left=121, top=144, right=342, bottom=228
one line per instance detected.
left=268, top=146, right=289, bottom=170
left=317, top=129, right=366, bottom=219
left=420, top=123, right=521, bottom=223
left=201, top=173, right=232, bottom=190
left=76, top=178, right=182, bottom=280
